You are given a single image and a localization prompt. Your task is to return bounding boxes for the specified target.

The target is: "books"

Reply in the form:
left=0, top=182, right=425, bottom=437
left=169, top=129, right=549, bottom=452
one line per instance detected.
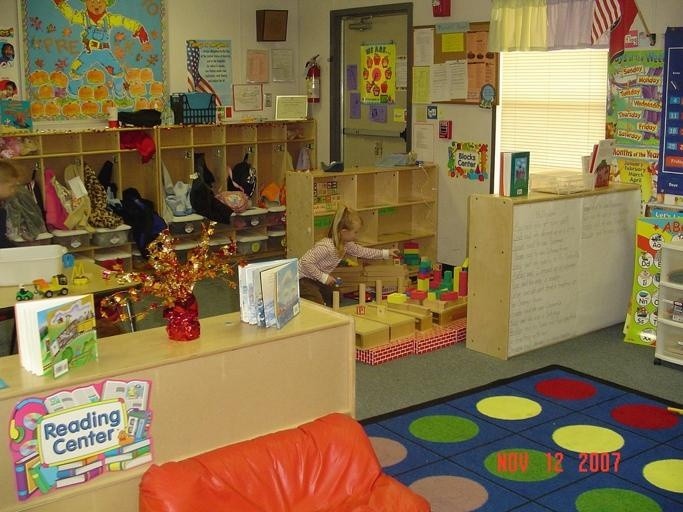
left=498, top=149, right=529, bottom=197
left=14, top=293, right=100, bottom=375
left=580, top=138, right=616, bottom=178
left=237, top=256, right=301, bottom=330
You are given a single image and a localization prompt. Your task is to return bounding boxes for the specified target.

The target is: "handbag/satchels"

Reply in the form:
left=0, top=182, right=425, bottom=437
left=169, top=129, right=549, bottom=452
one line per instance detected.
left=194, top=153, right=216, bottom=188
left=216, top=191, right=248, bottom=213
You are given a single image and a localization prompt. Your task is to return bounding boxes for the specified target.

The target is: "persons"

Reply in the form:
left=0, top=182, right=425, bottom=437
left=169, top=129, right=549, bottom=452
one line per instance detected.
left=0, top=44, right=13, bottom=65
left=0, top=83, right=13, bottom=98
left=0, top=164, right=20, bottom=247
left=296, top=204, right=405, bottom=305
left=52, top=1, right=149, bottom=107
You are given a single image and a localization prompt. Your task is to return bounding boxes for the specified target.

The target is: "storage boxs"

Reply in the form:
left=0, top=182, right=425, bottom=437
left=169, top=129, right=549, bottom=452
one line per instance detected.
left=209, top=212, right=235, bottom=234
left=209, top=236, right=232, bottom=259
left=96, top=249, right=132, bottom=272
left=54, top=225, right=90, bottom=250
left=174, top=241, right=203, bottom=264
left=270, top=229, right=287, bottom=250
left=269, top=204, right=287, bottom=226
left=163, top=211, right=204, bottom=238
left=94, top=222, right=133, bottom=248
left=72, top=255, right=95, bottom=267
left=10, top=232, right=54, bottom=247
left=237, top=233, right=267, bottom=257
left=235, top=207, right=268, bottom=232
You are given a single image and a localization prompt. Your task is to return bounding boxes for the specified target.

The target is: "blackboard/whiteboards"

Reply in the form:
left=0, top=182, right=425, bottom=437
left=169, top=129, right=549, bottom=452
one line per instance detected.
left=509, top=189, right=644, bottom=357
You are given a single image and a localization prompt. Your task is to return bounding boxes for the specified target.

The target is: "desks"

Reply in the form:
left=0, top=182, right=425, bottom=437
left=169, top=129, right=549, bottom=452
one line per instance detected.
left=1, top=248, right=142, bottom=357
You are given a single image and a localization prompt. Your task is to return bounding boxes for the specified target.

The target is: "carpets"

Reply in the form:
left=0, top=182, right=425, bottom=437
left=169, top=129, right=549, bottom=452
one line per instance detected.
left=355, top=365, right=681, bottom=510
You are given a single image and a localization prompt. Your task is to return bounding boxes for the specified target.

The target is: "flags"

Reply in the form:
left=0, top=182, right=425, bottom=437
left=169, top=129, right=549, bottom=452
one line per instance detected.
left=590, top=1, right=638, bottom=64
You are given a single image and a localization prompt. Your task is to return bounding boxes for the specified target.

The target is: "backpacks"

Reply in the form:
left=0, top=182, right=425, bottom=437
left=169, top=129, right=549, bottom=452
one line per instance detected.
left=228, top=162, right=256, bottom=198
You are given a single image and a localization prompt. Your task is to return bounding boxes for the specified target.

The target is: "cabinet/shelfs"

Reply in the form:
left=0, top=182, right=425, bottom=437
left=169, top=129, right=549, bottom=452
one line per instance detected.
left=157, top=119, right=318, bottom=267
left=285, top=163, right=440, bottom=282
left=652, top=241, right=683, bottom=370
left=0, top=117, right=157, bottom=277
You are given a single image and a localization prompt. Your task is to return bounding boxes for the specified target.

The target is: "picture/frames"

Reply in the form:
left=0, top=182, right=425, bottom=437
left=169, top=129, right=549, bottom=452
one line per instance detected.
left=274, top=95, right=307, bottom=120
left=231, top=83, right=263, bottom=112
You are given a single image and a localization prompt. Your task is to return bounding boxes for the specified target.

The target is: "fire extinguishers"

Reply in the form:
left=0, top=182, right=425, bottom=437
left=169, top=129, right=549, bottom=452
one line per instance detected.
left=305, top=54, right=320, bottom=103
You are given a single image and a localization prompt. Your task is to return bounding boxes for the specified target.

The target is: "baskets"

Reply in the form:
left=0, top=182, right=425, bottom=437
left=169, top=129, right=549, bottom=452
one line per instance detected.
left=530, top=169, right=596, bottom=195
left=169, top=94, right=216, bottom=125
